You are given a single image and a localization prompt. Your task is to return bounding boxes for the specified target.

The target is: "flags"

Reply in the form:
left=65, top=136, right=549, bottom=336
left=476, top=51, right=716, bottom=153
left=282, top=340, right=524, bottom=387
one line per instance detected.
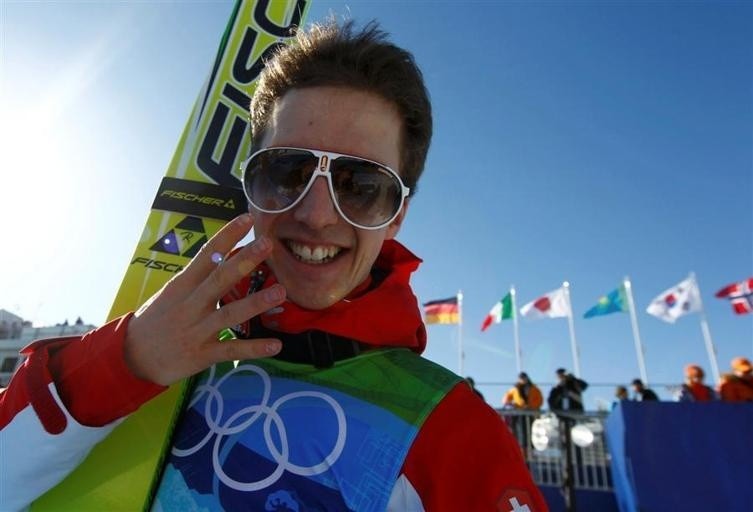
left=583, top=287, right=630, bottom=318
left=520, top=287, right=567, bottom=318
left=645, top=274, right=701, bottom=324
left=480, top=291, right=514, bottom=333
left=422, top=296, right=458, bottom=325
left=713, top=277, right=753, bottom=315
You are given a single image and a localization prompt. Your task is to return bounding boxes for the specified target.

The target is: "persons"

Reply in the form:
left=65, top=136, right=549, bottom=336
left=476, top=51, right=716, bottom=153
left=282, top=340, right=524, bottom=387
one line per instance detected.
left=547, top=368, right=588, bottom=453
left=501, top=372, right=544, bottom=452
left=611, top=386, right=629, bottom=409
left=631, top=378, right=659, bottom=402
left=678, top=364, right=718, bottom=403
left=716, top=358, right=753, bottom=403
left=0, top=13, right=549, bottom=512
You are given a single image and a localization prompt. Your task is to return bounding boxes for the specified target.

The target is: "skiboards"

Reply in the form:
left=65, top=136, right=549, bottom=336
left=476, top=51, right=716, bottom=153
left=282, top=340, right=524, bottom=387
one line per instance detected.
left=23, top=0, right=309, bottom=511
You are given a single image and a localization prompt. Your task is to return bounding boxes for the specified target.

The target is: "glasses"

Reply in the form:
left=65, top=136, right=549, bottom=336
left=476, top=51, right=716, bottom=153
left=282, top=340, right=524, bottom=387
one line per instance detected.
left=238, top=146, right=412, bottom=232
